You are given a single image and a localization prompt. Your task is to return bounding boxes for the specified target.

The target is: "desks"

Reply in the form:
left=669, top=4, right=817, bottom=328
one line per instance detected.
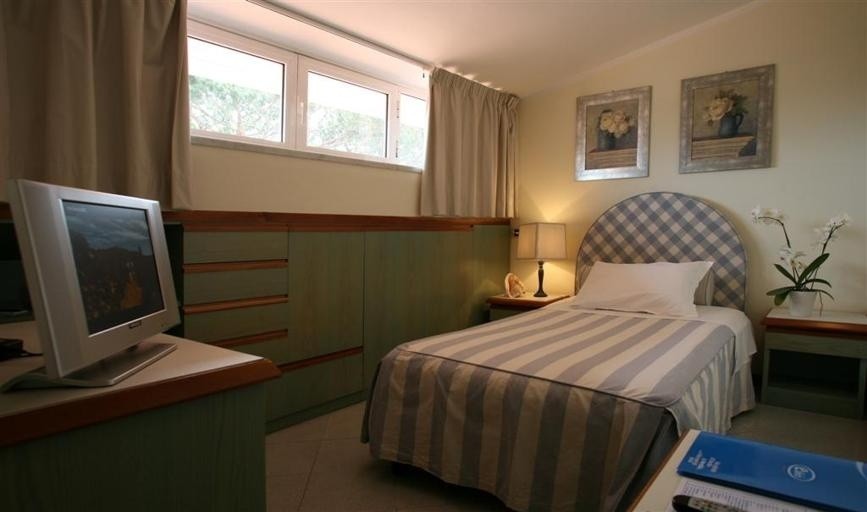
left=0, top=320, right=284, bottom=512
left=627, top=428, right=867, bottom=512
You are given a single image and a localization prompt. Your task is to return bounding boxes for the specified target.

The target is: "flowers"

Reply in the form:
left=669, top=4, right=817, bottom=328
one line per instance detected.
left=751, top=203, right=854, bottom=306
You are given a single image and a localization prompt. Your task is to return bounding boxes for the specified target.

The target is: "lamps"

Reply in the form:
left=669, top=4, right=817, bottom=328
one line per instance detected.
left=516, top=222, right=568, bottom=299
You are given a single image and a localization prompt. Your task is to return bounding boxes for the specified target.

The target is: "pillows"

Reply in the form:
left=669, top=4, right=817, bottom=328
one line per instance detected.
left=570, top=259, right=715, bottom=320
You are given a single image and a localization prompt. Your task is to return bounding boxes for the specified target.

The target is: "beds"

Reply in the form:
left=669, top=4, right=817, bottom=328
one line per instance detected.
left=359, top=190, right=761, bottom=512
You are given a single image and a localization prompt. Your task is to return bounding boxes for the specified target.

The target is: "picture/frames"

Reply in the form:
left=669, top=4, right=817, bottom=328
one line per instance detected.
left=679, top=64, right=775, bottom=173
left=576, top=86, right=651, bottom=181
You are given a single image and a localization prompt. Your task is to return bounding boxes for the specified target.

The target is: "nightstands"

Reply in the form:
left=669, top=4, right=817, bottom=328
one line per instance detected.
left=486, top=292, right=570, bottom=319
left=760, top=309, right=866, bottom=419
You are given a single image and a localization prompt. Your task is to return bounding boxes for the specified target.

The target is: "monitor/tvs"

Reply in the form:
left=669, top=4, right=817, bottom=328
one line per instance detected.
left=1, top=177, right=182, bottom=389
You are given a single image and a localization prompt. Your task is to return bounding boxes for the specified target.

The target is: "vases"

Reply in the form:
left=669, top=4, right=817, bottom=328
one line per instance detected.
left=789, top=290, right=817, bottom=318
